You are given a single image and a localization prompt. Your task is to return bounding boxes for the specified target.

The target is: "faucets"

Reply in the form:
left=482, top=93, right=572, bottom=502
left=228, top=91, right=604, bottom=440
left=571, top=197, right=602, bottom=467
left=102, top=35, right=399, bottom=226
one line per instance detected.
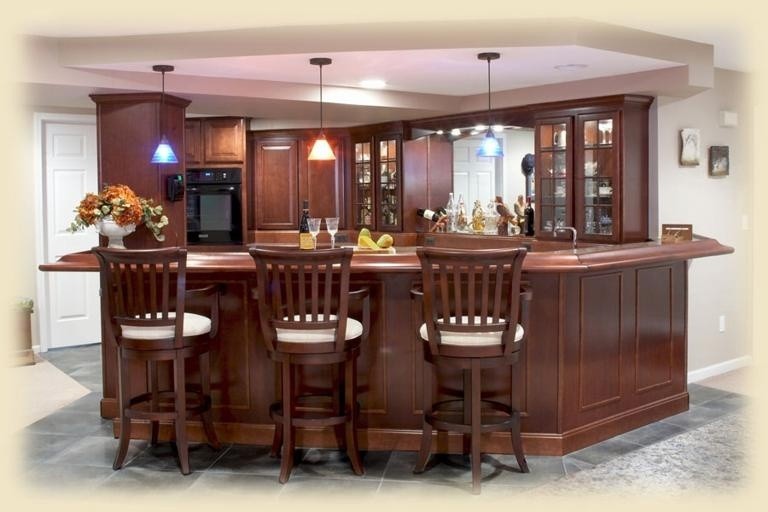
left=552, top=226, right=578, bottom=250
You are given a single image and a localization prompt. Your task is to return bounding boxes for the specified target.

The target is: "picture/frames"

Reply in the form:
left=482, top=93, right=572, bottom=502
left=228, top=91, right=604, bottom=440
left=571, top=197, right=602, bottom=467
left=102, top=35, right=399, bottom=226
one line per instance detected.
left=709, top=146, right=729, bottom=177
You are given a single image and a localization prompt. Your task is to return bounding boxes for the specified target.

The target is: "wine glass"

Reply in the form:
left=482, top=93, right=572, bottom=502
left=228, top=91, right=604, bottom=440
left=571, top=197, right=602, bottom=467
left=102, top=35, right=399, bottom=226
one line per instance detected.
left=308, top=217, right=322, bottom=248
left=598, top=120, right=613, bottom=146
left=324, top=218, right=341, bottom=250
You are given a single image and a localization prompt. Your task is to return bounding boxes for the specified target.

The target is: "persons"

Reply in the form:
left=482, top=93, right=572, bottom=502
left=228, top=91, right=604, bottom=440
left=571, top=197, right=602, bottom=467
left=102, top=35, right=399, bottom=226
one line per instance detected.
left=471, top=199, right=485, bottom=231
left=514, top=195, right=529, bottom=234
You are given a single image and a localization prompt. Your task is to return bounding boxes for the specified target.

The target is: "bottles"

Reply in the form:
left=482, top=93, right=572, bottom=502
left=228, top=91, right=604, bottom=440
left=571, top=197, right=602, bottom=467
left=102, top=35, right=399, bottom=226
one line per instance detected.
left=525, top=197, right=534, bottom=236
left=300, top=198, right=308, bottom=253
left=418, top=193, right=498, bottom=235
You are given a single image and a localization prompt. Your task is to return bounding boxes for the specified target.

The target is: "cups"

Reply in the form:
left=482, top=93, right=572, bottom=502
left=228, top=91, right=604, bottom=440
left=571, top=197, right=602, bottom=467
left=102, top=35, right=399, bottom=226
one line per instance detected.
left=599, top=185, right=613, bottom=198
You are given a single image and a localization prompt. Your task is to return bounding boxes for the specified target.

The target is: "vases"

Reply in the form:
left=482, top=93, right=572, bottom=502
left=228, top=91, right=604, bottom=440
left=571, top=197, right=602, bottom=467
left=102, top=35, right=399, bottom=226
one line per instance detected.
left=87, top=215, right=141, bottom=253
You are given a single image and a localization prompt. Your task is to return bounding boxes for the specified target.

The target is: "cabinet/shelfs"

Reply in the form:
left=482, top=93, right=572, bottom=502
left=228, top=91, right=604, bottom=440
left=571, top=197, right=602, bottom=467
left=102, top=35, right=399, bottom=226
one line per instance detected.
left=181, top=114, right=255, bottom=170
left=350, top=125, right=459, bottom=234
left=243, top=127, right=355, bottom=236
left=89, top=91, right=192, bottom=255
left=532, top=91, right=656, bottom=246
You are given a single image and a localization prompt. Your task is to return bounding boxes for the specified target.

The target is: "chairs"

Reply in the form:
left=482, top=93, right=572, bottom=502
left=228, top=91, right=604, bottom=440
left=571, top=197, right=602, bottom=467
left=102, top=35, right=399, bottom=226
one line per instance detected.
left=403, top=242, right=533, bottom=496
left=244, top=242, right=378, bottom=484
left=90, top=247, right=223, bottom=477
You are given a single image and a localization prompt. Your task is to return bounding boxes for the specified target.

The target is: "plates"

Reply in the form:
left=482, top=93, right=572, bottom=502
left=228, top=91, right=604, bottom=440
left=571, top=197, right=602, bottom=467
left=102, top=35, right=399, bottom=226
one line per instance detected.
left=554, top=178, right=566, bottom=198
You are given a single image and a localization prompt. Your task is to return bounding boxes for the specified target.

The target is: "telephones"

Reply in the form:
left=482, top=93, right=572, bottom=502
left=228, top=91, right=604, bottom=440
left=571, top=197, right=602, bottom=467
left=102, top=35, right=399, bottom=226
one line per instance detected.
left=167, top=174, right=184, bottom=202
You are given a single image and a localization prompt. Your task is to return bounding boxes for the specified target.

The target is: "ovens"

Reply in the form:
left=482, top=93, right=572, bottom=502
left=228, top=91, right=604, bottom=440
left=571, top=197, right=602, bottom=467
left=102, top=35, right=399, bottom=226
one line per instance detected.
left=186, top=186, right=240, bottom=241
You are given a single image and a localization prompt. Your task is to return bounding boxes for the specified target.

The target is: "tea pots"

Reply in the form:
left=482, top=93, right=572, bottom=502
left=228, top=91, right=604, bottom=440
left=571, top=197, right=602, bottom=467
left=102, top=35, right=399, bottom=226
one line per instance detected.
left=542, top=210, right=613, bottom=237
left=553, top=128, right=567, bottom=147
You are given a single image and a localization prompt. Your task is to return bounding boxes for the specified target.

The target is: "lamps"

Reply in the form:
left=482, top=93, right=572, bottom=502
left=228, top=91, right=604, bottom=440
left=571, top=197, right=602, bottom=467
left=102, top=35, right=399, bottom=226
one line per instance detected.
left=306, top=54, right=337, bottom=161
left=142, top=63, right=180, bottom=165
left=475, top=49, right=506, bottom=161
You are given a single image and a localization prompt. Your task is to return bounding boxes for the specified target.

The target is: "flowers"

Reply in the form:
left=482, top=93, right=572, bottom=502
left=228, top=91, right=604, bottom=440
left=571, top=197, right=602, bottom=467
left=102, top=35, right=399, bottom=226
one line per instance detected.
left=57, top=180, right=172, bottom=243
